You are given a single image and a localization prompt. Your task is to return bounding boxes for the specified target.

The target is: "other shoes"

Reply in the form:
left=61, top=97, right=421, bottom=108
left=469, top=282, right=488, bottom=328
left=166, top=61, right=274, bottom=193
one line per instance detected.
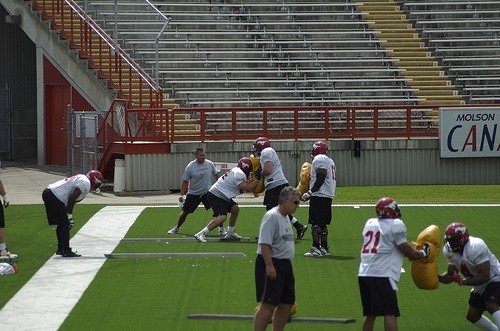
left=193, top=230, right=208, bottom=243
left=320, top=246, right=330, bottom=256
left=304, top=246, right=322, bottom=257
left=55, top=248, right=82, bottom=258
left=1, top=250, right=19, bottom=260
left=296, top=224, right=308, bottom=241
left=167, top=226, right=181, bottom=234
left=218, top=227, right=226, bottom=234
left=224, top=230, right=242, bottom=241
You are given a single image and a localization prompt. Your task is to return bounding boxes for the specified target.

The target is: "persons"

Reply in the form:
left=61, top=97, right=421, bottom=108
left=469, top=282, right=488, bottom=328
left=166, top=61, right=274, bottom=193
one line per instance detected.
left=358, top=197, right=430, bottom=331
left=253, top=186, right=300, bottom=331
left=0, top=180, right=17, bottom=261
left=194, top=157, right=261, bottom=243
left=303, top=141, right=336, bottom=257
left=168, top=149, right=221, bottom=234
left=253, top=137, right=308, bottom=239
left=438, top=221, right=500, bottom=331
left=42, top=170, right=104, bottom=257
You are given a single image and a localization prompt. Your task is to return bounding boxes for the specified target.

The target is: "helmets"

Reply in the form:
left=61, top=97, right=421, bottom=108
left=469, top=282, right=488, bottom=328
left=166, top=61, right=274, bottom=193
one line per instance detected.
left=86, top=170, right=104, bottom=191
left=375, top=197, right=400, bottom=218
left=237, top=156, right=252, bottom=175
left=312, top=140, right=329, bottom=155
left=445, top=223, right=469, bottom=243
left=253, top=137, right=270, bottom=152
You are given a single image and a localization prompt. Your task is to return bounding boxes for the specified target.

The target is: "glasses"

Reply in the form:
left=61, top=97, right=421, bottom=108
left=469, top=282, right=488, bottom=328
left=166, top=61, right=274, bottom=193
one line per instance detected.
left=293, top=200, right=300, bottom=205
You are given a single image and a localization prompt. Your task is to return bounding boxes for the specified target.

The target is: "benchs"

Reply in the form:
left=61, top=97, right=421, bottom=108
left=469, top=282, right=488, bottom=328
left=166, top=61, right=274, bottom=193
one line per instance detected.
left=81, top=0, right=500, bottom=137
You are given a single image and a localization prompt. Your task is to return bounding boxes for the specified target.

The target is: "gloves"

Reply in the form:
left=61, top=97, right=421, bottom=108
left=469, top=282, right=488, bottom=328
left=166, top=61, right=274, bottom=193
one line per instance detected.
left=449, top=273, right=463, bottom=285
left=301, top=192, right=311, bottom=202
left=254, top=167, right=262, bottom=180
left=179, top=194, right=186, bottom=210
left=2, top=194, right=9, bottom=209
left=67, top=217, right=75, bottom=230
left=419, top=242, right=430, bottom=257
